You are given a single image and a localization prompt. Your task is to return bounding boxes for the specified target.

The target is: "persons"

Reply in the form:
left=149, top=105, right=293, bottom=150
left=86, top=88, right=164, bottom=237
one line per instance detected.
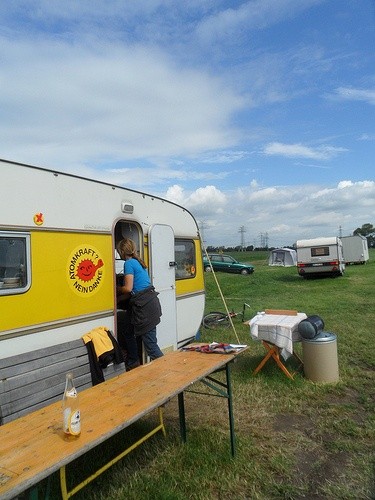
left=117, top=238, right=162, bottom=366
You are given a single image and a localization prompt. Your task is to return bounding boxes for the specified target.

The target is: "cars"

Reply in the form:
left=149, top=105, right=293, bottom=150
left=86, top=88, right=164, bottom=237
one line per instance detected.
left=202, top=254, right=255, bottom=275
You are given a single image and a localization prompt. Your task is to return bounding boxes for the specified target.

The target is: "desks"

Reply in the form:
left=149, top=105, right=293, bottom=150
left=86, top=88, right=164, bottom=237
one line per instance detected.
left=244, top=312, right=308, bottom=381
left=0, top=343, right=250, bottom=500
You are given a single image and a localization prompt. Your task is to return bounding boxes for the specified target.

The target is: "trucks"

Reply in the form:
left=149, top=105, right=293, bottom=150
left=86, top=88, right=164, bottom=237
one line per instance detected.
left=295, top=237, right=347, bottom=278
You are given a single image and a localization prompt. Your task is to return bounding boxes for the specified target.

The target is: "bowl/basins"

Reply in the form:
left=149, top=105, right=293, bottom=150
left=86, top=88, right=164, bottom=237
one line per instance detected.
left=2, top=278, right=19, bottom=283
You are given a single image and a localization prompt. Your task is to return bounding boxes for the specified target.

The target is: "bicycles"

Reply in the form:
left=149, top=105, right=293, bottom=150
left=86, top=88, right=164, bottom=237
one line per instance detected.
left=202, top=303, right=251, bottom=329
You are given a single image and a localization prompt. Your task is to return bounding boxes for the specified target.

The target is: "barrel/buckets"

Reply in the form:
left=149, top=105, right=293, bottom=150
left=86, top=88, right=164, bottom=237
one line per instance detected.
left=301, top=332, right=339, bottom=384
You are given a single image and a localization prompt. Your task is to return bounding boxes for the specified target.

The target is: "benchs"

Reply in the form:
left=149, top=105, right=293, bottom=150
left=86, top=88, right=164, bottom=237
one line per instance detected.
left=0, top=327, right=166, bottom=499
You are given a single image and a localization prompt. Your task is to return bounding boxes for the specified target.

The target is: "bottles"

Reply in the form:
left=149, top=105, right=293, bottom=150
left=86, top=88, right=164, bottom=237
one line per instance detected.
left=63, top=373, right=80, bottom=440
